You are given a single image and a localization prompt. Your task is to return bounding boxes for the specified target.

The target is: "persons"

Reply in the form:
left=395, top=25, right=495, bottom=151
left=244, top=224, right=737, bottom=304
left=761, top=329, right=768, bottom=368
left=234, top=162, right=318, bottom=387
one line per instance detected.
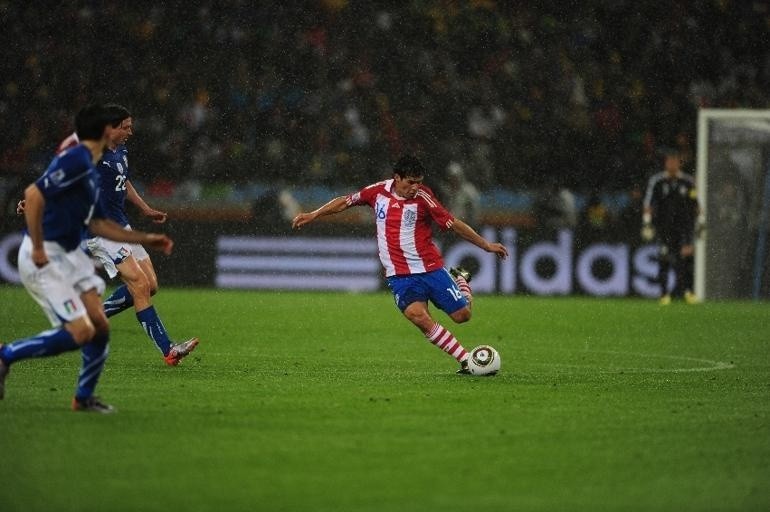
left=79, top=104, right=199, bottom=367
left=0, top=106, right=174, bottom=411
left=288, top=150, right=508, bottom=375
left=640, top=147, right=697, bottom=307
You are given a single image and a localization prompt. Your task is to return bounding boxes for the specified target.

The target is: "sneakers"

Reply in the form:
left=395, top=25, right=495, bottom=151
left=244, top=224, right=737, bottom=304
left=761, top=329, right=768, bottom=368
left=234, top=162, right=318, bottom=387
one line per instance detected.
left=456, top=359, right=472, bottom=375
left=73, top=395, right=114, bottom=416
left=450, top=266, right=473, bottom=284
left=163, top=336, right=200, bottom=366
left=0, top=343, right=10, bottom=401
left=661, top=291, right=695, bottom=305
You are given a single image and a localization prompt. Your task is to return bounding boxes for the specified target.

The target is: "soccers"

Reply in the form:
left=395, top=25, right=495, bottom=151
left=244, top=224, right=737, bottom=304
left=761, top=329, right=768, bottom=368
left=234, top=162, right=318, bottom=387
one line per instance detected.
left=468, top=345, right=500, bottom=376
